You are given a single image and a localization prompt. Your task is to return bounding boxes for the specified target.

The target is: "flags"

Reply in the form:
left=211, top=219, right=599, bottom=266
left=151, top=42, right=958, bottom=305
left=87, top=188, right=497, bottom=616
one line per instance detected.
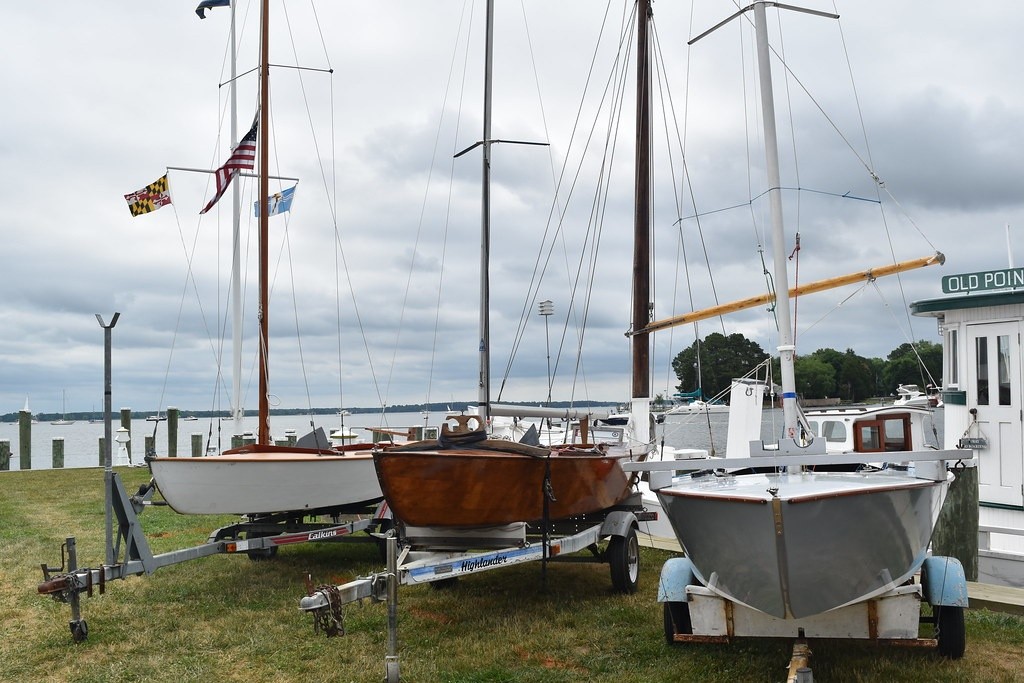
left=124, top=174, right=172, bottom=217
left=199, top=119, right=259, bottom=214
left=254, top=185, right=296, bottom=217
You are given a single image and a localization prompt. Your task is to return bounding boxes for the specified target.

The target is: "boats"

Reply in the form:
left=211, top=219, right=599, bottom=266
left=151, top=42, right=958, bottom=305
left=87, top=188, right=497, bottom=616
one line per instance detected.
left=336, top=410, right=351, bottom=416
left=465, top=405, right=725, bottom=539
left=183, top=415, right=199, bottom=421
left=664, top=396, right=729, bottom=414
left=222, top=416, right=233, bottom=420
left=144, top=416, right=166, bottom=420
left=598, top=402, right=666, bottom=424
left=892, top=382, right=940, bottom=409
left=768, top=402, right=941, bottom=467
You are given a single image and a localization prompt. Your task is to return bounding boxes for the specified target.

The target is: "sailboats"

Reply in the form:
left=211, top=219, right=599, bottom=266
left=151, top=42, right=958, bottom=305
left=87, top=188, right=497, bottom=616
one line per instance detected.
left=420, top=393, right=431, bottom=414
left=370, top=0, right=651, bottom=529
left=445, top=393, right=457, bottom=414
left=140, top=0, right=384, bottom=517
left=50, top=388, right=76, bottom=425
left=87, top=398, right=105, bottom=423
left=650, top=0, right=975, bottom=619
left=13, top=394, right=39, bottom=425
